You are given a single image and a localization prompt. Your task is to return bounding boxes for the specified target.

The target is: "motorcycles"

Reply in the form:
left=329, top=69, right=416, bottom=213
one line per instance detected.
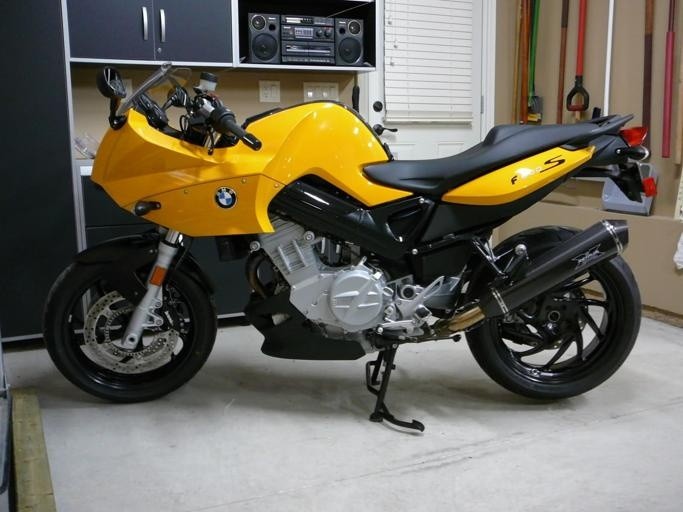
left=41, top=62, right=656, bottom=432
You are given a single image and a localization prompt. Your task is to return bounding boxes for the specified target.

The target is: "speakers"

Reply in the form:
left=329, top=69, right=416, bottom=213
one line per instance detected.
left=335, top=17, right=363, bottom=65
left=245, top=11, right=282, bottom=63
